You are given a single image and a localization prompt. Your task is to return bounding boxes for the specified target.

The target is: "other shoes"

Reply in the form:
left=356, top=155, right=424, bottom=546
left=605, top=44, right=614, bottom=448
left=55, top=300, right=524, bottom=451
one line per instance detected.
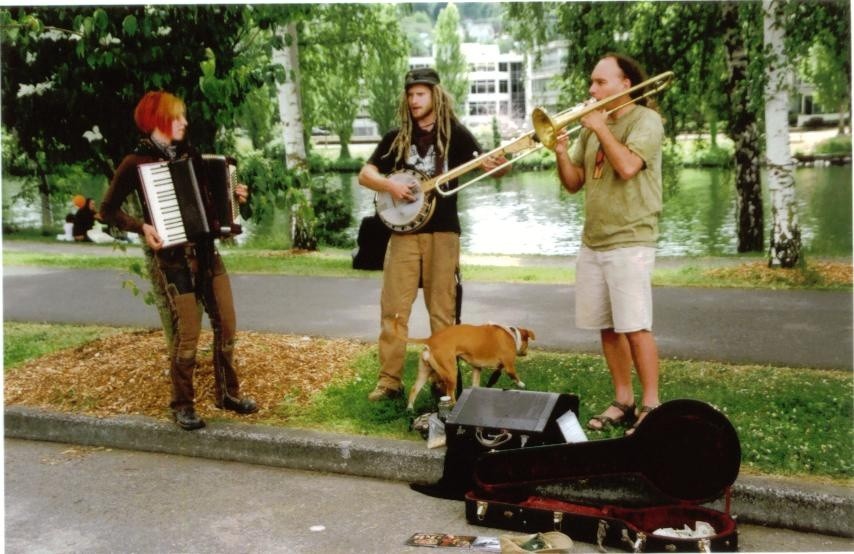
left=368, top=386, right=398, bottom=401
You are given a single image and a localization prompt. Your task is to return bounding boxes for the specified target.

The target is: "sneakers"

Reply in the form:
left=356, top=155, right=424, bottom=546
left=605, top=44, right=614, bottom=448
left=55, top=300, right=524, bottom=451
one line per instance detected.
left=226, top=397, right=259, bottom=413
left=175, top=409, right=201, bottom=428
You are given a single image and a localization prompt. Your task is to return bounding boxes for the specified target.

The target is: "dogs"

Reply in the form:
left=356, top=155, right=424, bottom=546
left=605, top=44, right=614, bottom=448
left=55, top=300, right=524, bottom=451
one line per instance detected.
left=406, top=321, right=536, bottom=412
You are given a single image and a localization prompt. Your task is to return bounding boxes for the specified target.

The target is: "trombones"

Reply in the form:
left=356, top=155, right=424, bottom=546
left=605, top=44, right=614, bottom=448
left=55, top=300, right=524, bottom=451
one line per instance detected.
left=436, top=72, right=676, bottom=197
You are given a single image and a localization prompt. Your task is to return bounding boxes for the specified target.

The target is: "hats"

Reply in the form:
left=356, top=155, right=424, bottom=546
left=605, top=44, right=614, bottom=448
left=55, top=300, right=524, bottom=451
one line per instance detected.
left=404, top=68, right=440, bottom=85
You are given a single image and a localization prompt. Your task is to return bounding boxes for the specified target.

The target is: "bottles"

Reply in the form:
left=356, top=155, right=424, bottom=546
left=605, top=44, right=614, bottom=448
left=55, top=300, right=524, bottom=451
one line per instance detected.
left=438, top=396, right=454, bottom=424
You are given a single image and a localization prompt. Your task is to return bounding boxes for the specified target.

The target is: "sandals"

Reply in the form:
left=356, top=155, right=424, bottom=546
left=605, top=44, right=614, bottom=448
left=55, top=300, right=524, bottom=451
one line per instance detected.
left=624, top=406, right=652, bottom=437
left=586, top=401, right=636, bottom=430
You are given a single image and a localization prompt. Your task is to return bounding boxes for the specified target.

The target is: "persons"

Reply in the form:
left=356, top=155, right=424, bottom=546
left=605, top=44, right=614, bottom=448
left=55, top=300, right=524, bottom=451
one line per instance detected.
left=359, top=69, right=512, bottom=401
left=69, top=194, right=104, bottom=241
left=552, top=53, right=664, bottom=436
left=99, top=91, right=260, bottom=430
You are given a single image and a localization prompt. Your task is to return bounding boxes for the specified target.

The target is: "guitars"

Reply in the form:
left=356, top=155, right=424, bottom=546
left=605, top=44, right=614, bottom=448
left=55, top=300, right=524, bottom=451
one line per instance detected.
left=375, top=129, right=566, bottom=234
